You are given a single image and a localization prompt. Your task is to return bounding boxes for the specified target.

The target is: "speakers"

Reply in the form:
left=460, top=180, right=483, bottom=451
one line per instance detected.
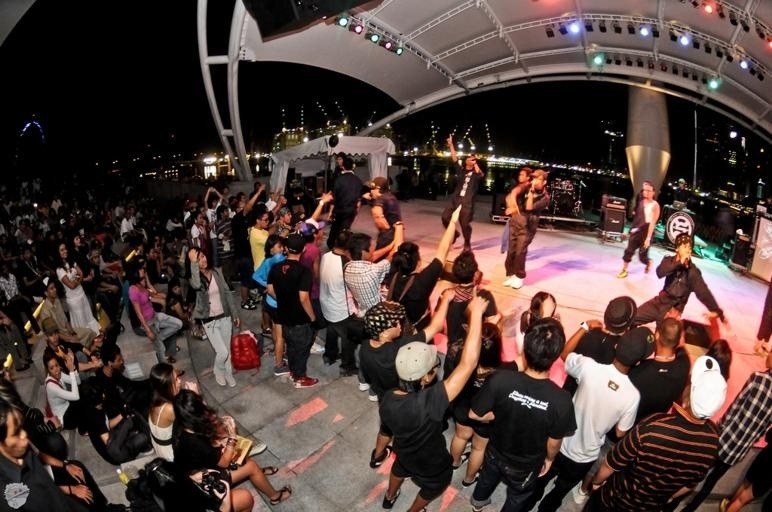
left=732, top=233, right=751, bottom=268
left=599, top=206, right=626, bottom=233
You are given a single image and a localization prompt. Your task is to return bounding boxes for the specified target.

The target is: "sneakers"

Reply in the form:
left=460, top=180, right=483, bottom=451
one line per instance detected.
left=168, top=347, right=185, bottom=376
left=645, top=262, right=653, bottom=273
left=215, top=374, right=226, bottom=386
left=617, top=271, right=628, bottom=279
left=246, top=444, right=266, bottom=458
left=512, top=278, right=524, bottom=288
left=503, top=275, right=516, bottom=288
left=293, top=375, right=319, bottom=388
left=370, top=446, right=392, bottom=467
left=241, top=299, right=257, bottom=309
left=325, top=353, right=378, bottom=401
left=451, top=452, right=492, bottom=512
left=272, top=365, right=290, bottom=376
left=383, top=488, right=401, bottom=508
left=310, top=344, right=324, bottom=357
left=572, top=481, right=588, bottom=503
left=225, top=376, right=236, bottom=387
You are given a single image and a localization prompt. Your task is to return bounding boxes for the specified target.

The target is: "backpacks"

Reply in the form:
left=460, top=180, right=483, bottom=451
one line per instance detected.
left=231, top=330, right=261, bottom=370
left=258, top=333, right=286, bottom=357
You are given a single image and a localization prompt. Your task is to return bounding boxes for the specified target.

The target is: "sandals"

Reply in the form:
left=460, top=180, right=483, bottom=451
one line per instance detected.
left=260, top=466, right=279, bottom=476
left=271, top=486, right=292, bottom=505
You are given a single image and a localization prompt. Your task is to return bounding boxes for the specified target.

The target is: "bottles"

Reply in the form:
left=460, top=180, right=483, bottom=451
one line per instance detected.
left=115, top=462, right=140, bottom=485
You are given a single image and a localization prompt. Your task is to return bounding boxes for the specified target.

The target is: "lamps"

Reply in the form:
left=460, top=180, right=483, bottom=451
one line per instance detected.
left=679, top=0, right=772, bottom=47
left=426, top=61, right=431, bottom=71
left=545, top=13, right=768, bottom=81
left=335, top=13, right=404, bottom=55
left=591, top=50, right=721, bottom=89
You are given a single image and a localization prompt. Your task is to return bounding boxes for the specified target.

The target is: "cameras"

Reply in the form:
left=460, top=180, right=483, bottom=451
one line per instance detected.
left=203, top=471, right=225, bottom=494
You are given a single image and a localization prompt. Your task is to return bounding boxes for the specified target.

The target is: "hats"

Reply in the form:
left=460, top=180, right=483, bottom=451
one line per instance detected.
left=394, top=342, right=438, bottom=381
left=42, top=318, right=58, bottom=335
left=91, top=239, right=102, bottom=247
left=374, top=176, right=388, bottom=188
left=299, top=219, right=325, bottom=237
left=287, top=234, right=305, bottom=253
left=617, top=326, right=657, bottom=363
left=530, top=169, right=548, bottom=178
left=86, top=250, right=99, bottom=260
left=690, top=355, right=727, bottom=420
left=604, top=297, right=637, bottom=331
left=363, top=302, right=406, bottom=336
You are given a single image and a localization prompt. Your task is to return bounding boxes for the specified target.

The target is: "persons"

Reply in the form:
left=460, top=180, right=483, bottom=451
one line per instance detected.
left=0, top=131, right=772, bottom=512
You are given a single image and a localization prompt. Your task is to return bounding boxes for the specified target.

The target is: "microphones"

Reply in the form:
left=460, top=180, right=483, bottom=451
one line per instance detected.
left=525, top=181, right=533, bottom=186
left=360, top=193, right=373, bottom=199
left=471, top=158, right=477, bottom=161
left=685, top=259, right=689, bottom=268
